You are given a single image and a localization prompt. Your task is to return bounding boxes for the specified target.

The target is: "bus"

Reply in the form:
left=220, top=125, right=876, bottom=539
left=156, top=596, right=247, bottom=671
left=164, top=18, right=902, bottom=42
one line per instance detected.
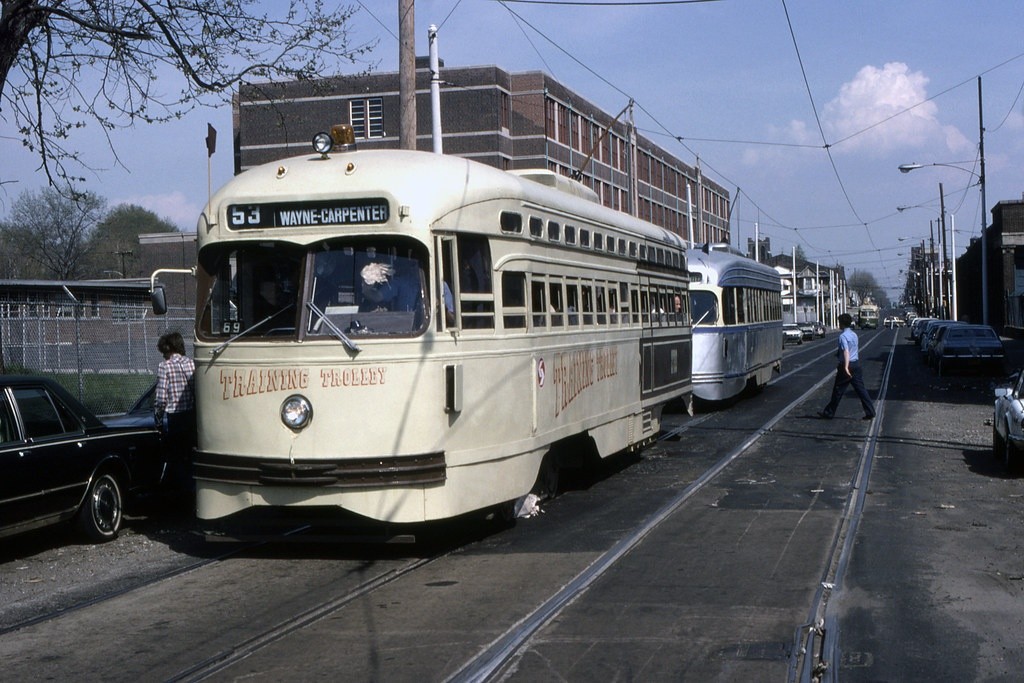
left=679, top=240, right=784, bottom=407
left=149, top=124, right=695, bottom=544
left=859, top=305, right=880, bottom=330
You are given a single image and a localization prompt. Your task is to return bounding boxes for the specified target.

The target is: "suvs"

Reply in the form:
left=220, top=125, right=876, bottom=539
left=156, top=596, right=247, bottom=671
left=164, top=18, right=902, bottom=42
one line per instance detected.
left=883, top=316, right=907, bottom=328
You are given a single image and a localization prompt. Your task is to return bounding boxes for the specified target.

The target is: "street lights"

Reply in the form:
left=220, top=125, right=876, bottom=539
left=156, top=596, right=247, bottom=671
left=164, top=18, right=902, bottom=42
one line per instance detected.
left=896, top=205, right=951, bottom=321
left=900, top=162, right=988, bottom=327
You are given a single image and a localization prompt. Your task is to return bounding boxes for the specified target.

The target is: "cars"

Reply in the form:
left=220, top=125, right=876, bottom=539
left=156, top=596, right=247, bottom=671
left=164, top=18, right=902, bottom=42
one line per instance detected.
left=851, top=313, right=860, bottom=329
left=904, top=307, right=964, bottom=365
left=783, top=324, right=803, bottom=346
left=813, top=322, right=826, bottom=339
left=938, top=324, right=1003, bottom=378
left=991, top=363, right=1024, bottom=468
left=797, top=321, right=816, bottom=340
left=98, top=377, right=167, bottom=444
left=0, top=374, right=161, bottom=544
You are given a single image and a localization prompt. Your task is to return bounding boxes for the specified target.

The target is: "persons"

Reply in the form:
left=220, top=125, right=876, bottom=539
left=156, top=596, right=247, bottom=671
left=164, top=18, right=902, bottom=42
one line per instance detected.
left=549, top=294, right=682, bottom=326
left=153, top=333, right=195, bottom=516
left=444, top=239, right=489, bottom=327
left=362, top=247, right=454, bottom=329
left=817, top=313, right=876, bottom=420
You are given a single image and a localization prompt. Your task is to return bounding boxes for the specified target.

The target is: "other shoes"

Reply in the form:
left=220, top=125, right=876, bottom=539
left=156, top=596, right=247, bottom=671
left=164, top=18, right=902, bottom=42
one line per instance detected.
left=862, top=412, right=876, bottom=420
left=816, top=411, right=833, bottom=420
